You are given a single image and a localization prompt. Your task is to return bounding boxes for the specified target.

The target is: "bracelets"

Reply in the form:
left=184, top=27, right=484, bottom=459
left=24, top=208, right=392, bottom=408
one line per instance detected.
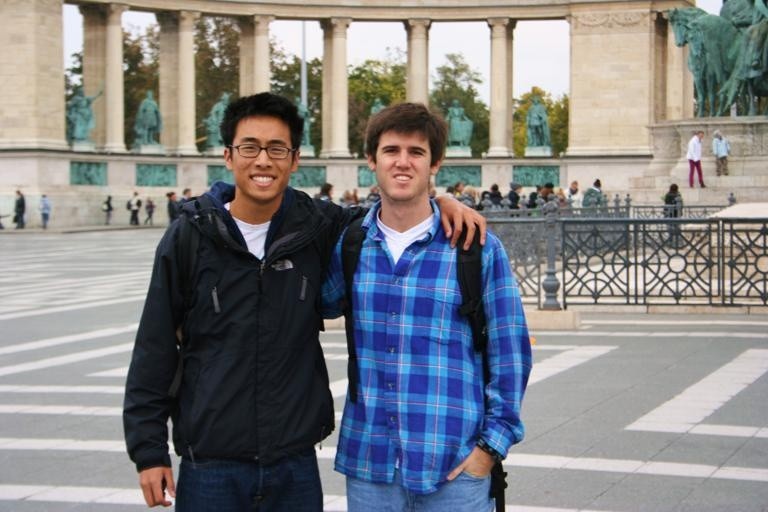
left=476, top=437, right=500, bottom=458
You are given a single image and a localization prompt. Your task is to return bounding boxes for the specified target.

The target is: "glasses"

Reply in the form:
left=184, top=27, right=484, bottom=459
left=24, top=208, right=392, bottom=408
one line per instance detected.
left=227, top=142, right=297, bottom=160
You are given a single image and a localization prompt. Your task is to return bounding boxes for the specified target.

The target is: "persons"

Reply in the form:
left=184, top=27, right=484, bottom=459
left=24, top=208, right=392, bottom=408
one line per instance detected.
left=210, top=92, right=230, bottom=142
left=442, top=98, right=472, bottom=146
left=447, top=179, right=604, bottom=214
left=686, top=131, right=706, bottom=188
left=13, top=191, right=26, bottom=229
left=712, top=129, right=731, bottom=176
left=102, top=195, right=113, bottom=225
left=166, top=189, right=190, bottom=223
left=134, top=88, right=159, bottom=146
left=526, top=96, right=550, bottom=146
left=317, top=103, right=533, bottom=512
left=66, top=84, right=101, bottom=144
left=145, top=197, right=156, bottom=227
left=663, top=184, right=683, bottom=241
left=316, top=183, right=381, bottom=207
left=122, top=91, right=486, bottom=512
left=126, top=192, right=141, bottom=225
left=38, top=195, right=51, bottom=230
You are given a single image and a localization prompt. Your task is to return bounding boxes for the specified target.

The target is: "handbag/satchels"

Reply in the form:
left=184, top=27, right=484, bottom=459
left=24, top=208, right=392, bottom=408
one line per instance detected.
left=13, top=215, right=18, bottom=222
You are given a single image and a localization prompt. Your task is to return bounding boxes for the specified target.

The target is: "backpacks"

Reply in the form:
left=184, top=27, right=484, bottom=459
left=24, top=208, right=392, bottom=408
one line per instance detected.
left=101, top=201, right=108, bottom=211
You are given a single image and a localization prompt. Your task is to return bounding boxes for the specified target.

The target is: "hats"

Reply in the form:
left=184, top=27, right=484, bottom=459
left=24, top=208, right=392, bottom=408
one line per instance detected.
left=509, top=181, right=521, bottom=191
left=594, top=179, right=601, bottom=188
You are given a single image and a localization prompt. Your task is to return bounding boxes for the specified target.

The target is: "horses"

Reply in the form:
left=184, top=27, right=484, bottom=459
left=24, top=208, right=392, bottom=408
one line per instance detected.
left=663, top=5, right=768, bottom=118
